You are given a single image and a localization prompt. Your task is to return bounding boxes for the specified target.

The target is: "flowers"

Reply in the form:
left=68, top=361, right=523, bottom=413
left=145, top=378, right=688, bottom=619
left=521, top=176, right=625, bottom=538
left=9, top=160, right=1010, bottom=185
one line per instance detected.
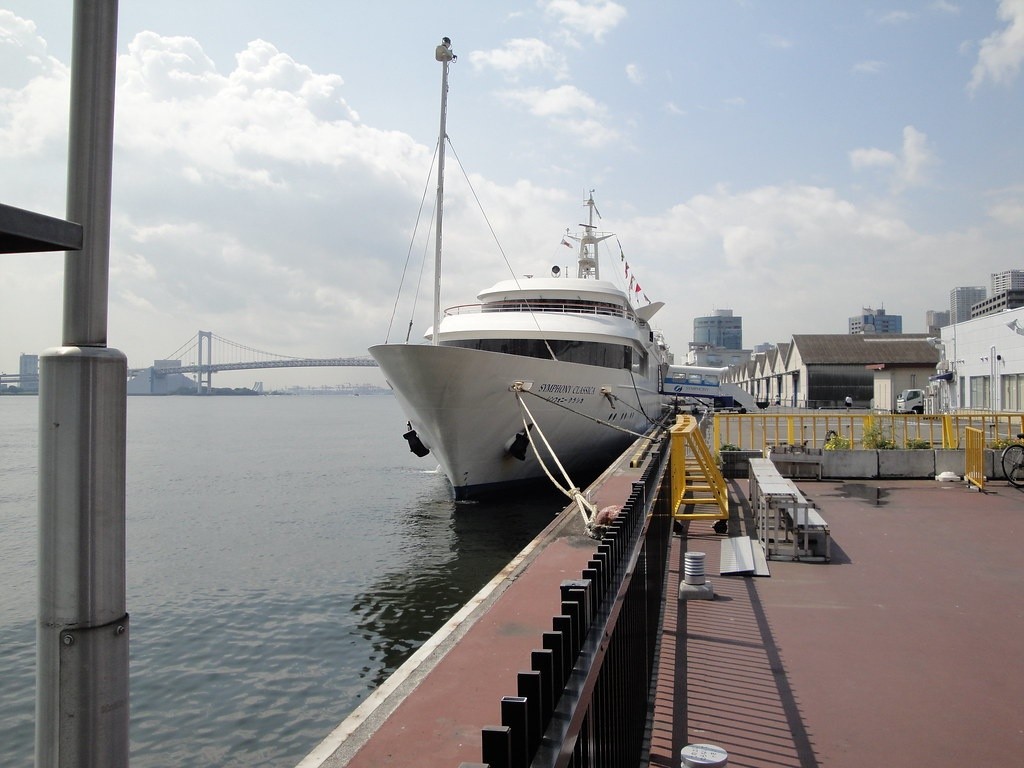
left=823, top=435, right=851, bottom=451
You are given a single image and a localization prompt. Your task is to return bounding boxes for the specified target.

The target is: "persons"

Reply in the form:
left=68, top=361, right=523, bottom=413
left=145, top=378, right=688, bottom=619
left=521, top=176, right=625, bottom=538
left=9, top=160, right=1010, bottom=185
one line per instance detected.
left=845, top=395, right=852, bottom=412
left=755, top=394, right=758, bottom=401
left=775, top=395, right=780, bottom=405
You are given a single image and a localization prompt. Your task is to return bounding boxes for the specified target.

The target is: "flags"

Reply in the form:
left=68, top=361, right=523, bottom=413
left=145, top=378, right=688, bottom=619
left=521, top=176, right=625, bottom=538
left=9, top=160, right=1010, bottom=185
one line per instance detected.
left=635, top=284, right=641, bottom=292
left=625, top=263, right=629, bottom=279
left=621, top=252, right=624, bottom=261
left=631, top=275, right=635, bottom=283
left=560, top=239, right=573, bottom=248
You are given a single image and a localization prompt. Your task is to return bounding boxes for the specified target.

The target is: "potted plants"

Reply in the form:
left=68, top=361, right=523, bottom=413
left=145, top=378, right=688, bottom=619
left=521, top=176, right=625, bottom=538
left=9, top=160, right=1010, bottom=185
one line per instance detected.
left=862, top=424, right=936, bottom=479
left=720, top=444, right=763, bottom=479
left=986, top=439, right=1024, bottom=478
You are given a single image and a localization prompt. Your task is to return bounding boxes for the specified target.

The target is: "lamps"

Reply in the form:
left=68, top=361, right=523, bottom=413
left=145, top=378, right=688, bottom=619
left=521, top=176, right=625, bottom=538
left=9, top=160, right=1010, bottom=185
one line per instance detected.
left=1003, top=319, right=1020, bottom=331
left=927, top=336, right=945, bottom=347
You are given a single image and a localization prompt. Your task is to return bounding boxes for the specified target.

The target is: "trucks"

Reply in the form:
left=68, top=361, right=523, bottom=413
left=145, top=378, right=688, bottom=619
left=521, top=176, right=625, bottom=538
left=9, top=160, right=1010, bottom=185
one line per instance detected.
left=895, top=388, right=923, bottom=414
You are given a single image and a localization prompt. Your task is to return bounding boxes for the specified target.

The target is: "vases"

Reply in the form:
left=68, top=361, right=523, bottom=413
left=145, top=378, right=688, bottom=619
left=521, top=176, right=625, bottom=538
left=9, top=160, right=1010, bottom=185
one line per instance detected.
left=766, top=446, right=820, bottom=478
left=932, top=447, right=993, bottom=478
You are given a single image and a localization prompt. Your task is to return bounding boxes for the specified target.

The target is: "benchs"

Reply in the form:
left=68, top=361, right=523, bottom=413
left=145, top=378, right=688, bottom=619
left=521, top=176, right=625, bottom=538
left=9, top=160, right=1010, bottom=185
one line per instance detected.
left=748, top=457, right=830, bottom=562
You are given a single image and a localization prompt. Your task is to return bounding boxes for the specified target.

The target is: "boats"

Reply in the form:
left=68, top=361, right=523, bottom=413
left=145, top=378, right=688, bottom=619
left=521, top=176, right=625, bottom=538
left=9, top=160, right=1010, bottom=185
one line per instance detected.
left=368, top=33, right=766, bottom=509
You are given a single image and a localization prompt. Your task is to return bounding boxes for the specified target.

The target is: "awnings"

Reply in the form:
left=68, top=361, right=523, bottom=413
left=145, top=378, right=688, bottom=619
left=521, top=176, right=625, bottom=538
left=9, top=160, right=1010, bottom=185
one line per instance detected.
left=929, top=373, right=953, bottom=380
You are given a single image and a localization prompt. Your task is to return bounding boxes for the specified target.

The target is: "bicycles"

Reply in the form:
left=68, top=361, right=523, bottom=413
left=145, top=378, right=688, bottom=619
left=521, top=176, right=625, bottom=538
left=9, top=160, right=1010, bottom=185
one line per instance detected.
left=999, top=433, right=1024, bottom=488
